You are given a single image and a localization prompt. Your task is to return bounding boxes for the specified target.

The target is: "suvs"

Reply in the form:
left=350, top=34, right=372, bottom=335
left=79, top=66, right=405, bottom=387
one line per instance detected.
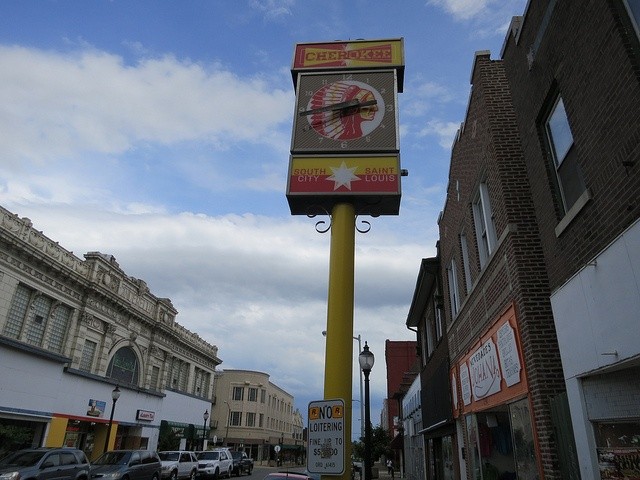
left=89, top=449, right=162, bottom=480
left=158, top=451, right=199, bottom=480
left=198, top=447, right=233, bottom=479
left=0, top=446, right=90, bottom=480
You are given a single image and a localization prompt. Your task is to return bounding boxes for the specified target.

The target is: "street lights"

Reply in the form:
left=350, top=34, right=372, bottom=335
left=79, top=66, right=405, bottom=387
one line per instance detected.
left=280, top=432, right=284, bottom=466
left=103, top=384, right=120, bottom=452
left=359, top=341, right=374, bottom=480
left=201, top=409, right=209, bottom=451
left=322, top=330, right=364, bottom=438
left=222, top=401, right=231, bottom=447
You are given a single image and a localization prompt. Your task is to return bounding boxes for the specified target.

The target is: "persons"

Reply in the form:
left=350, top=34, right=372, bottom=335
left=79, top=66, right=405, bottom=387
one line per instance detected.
left=387, top=456, right=392, bottom=477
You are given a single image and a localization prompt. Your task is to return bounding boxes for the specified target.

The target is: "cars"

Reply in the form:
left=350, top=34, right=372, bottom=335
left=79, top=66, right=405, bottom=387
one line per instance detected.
left=232, top=451, right=254, bottom=476
